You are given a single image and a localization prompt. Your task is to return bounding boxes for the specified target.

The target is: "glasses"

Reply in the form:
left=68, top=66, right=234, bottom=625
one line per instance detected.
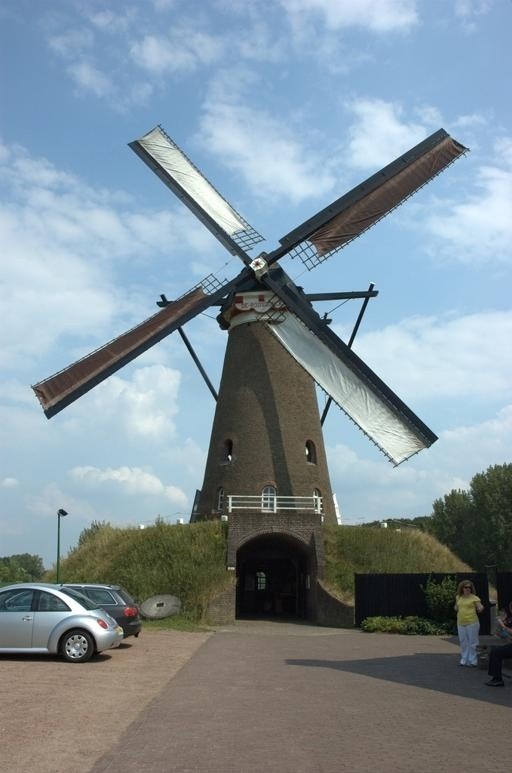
left=463, top=586, right=472, bottom=588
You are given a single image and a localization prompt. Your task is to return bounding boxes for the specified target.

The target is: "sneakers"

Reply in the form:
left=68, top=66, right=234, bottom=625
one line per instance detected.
left=485, top=679, right=505, bottom=686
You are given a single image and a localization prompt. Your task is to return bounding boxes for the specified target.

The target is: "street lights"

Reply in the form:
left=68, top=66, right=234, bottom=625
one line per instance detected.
left=57, top=509, right=68, bottom=584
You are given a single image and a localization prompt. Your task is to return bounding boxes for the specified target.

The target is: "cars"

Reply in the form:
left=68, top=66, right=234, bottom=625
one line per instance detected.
left=0, top=582, right=142, bottom=663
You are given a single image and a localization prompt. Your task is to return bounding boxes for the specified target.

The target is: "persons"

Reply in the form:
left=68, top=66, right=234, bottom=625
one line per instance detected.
left=452, top=578, right=486, bottom=667
left=494, top=607, right=511, bottom=642
left=484, top=600, right=511, bottom=687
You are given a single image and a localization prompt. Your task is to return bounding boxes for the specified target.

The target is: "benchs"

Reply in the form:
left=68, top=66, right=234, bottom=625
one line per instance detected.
left=476, top=646, right=512, bottom=679
